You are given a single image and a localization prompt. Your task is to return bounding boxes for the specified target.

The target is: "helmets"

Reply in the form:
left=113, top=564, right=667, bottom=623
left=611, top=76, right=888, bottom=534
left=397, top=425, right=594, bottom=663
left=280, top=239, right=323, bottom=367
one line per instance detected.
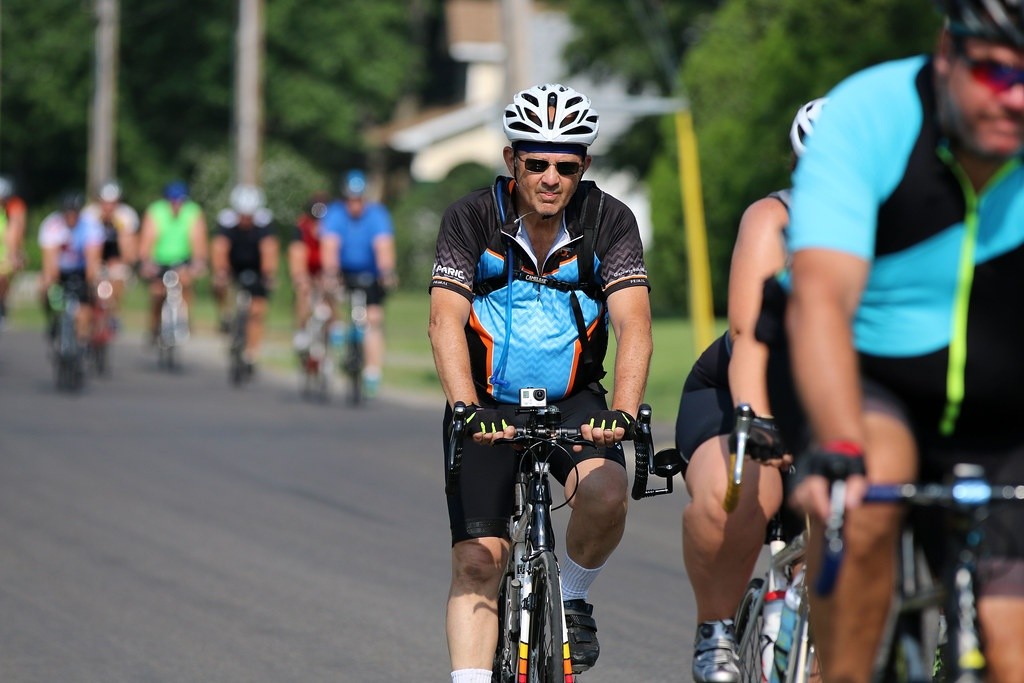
left=60, top=191, right=84, bottom=211
left=503, top=83, right=601, bottom=148
left=99, top=184, right=121, bottom=204
left=164, top=182, right=187, bottom=199
left=943, top=0, right=1024, bottom=50
left=230, top=184, right=267, bottom=214
left=788, top=100, right=824, bottom=156
left=342, top=171, right=367, bottom=196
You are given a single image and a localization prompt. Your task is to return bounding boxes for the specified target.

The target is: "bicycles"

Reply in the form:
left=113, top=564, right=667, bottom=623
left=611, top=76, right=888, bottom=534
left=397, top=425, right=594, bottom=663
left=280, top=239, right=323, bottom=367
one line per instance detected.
left=721, top=400, right=1024, bottom=683
left=35, top=269, right=398, bottom=409
left=441, top=398, right=685, bottom=683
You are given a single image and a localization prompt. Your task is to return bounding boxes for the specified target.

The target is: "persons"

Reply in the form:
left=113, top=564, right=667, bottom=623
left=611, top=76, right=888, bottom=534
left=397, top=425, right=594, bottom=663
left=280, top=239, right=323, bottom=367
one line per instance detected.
left=38, top=181, right=140, bottom=344
left=209, top=184, right=281, bottom=364
left=785, top=0, right=1024, bottom=683
left=288, top=168, right=397, bottom=392
left=138, top=181, right=209, bottom=333
left=428, top=82, right=654, bottom=683
left=0, top=177, right=28, bottom=316
left=674, top=98, right=827, bottom=683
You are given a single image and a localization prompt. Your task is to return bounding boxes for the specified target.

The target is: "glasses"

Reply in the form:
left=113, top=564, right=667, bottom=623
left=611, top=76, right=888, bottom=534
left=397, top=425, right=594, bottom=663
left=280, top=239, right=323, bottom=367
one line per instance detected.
left=951, top=37, right=1024, bottom=91
left=513, top=151, right=584, bottom=175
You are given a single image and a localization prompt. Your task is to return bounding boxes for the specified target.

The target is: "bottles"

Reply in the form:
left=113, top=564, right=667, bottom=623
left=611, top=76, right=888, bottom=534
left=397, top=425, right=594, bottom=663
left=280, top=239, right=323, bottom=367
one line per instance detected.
left=763, top=579, right=786, bottom=681
left=768, top=581, right=806, bottom=683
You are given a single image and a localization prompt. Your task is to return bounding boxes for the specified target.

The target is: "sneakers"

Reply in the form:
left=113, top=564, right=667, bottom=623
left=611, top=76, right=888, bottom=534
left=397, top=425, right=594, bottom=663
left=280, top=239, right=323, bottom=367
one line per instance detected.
left=691, top=619, right=741, bottom=683
left=564, top=595, right=599, bottom=667
